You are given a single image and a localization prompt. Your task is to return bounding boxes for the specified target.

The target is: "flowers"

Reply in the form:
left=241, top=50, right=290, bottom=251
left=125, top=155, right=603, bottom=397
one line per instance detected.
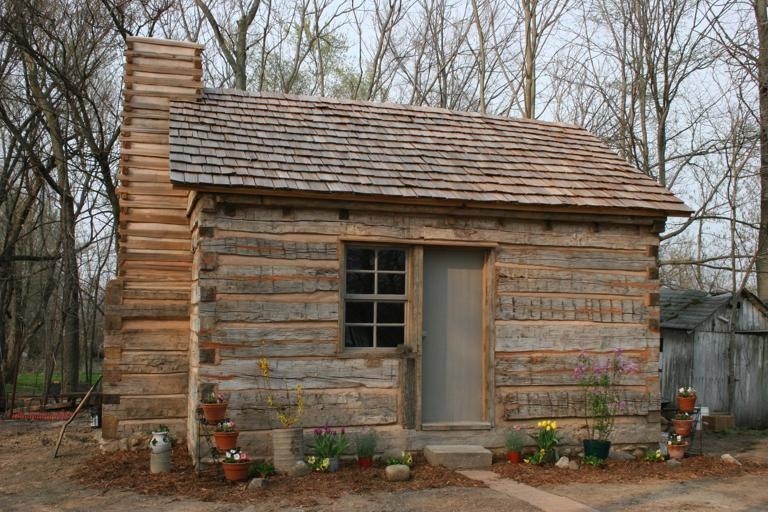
left=353, top=423, right=378, bottom=458
left=663, top=432, right=683, bottom=446
left=208, top=418, right=236, bottom=431
left=573, top=347, right=635, bottom=441
left=307, top=455, right=330, bottom=472
left=311, top=424, right=351, bottom=458
left=525, top=420, right=564, bottom=450
left=674, top=413, right=691, bottom=421
left=523, top=449, right=545, bottom=465
left=501, top=424, right=524, bottom=448
left=254, top=358, right=305, bottom=426
left=645, top=448, right=664, bottom=461
left=390, top=451, right=413, bottom=466
left=223, top=447, right=250, bottom=463
left=678, top=386, right=699, bottom=396
left=196, top=383, right=228, bottom=404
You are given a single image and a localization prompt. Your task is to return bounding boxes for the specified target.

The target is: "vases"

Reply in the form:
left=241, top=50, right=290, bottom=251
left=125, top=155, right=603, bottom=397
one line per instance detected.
left=664, top=443, right=689, bottom=458
left=272, top=428, right=304, bottom=474
left=507, top=449, right=522, bottom=464
left=676, top=395, right=697, bottom=412
left=213, top=430, right=239, bottom=451
left=318, top=458, right=339, bottom=473
left=536, top=449, right=553, bottom=462
left=582, top=440, right=611, bottom=463
left=672, top=417, right=695, bottom=435
left=201, top=403, right=228, bottom=423
left=353, top=457, right=376, bottom=468
left=223, top=460, right=253, bottom=482
